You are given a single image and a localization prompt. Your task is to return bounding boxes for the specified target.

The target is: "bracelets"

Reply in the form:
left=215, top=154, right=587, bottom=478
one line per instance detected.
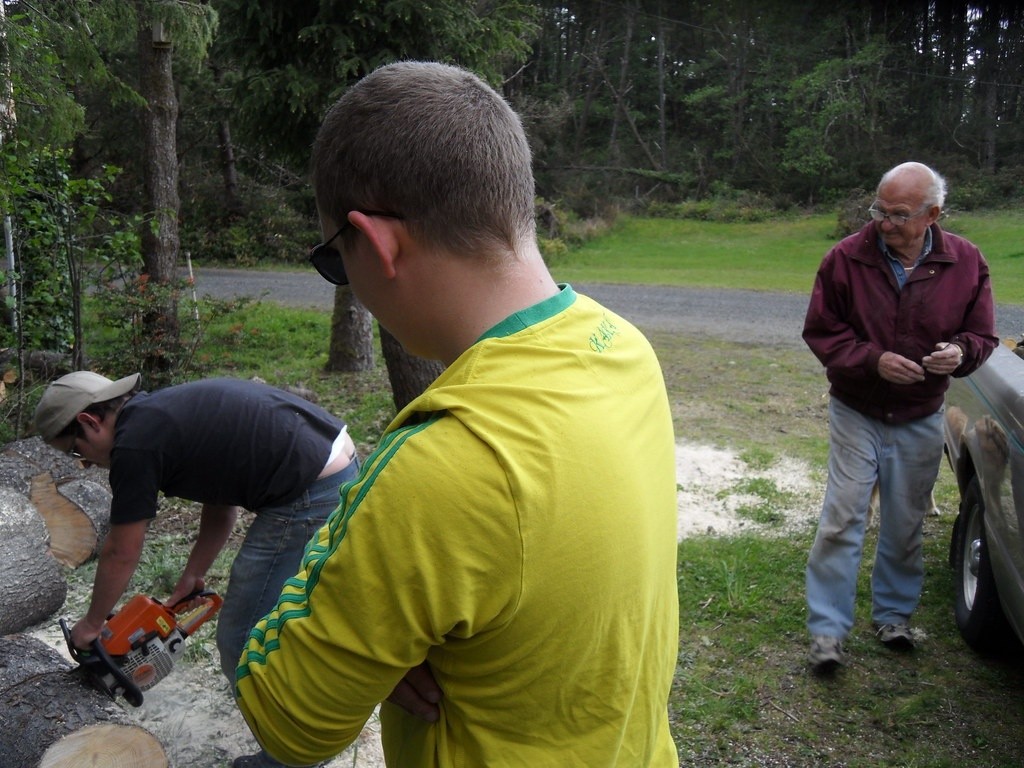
left=955, top=344, right=963, bottom=360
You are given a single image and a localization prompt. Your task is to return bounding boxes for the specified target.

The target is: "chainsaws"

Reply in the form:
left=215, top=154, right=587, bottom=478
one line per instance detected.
left=57, top=587, right=223, bottom=707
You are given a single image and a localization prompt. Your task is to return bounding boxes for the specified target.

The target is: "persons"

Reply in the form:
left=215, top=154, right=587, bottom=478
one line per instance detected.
left=33, top=369, right=361, bottom=767
left=227, top=57, right=684, bottom=768
left=799, top=159, right=1001, bottom=674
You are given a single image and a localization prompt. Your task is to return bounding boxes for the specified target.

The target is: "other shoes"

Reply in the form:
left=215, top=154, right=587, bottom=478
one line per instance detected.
left=233, top=750, right=334, bottom=768
left=808, top=638, right=842, bottom=671
left=872, top=621, right=915, bottom=648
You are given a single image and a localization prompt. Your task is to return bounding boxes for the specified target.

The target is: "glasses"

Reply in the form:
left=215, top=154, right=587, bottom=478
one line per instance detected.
left=868, top=200, right=929, bottom=225
left=67, top=426, right=86, bottom=460
left=309, top=212, right=409, bottom=285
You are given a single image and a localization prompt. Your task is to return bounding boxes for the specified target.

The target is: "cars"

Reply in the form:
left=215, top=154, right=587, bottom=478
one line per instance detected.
left=938, top=339, right=1023, bottom=670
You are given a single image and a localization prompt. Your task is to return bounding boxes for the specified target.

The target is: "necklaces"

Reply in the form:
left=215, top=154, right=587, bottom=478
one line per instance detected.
left=904, top=267, right=914, bottom=271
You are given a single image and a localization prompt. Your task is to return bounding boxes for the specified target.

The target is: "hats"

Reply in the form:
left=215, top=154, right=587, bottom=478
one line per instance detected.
left=36, top=370, right=143, bottom=443
